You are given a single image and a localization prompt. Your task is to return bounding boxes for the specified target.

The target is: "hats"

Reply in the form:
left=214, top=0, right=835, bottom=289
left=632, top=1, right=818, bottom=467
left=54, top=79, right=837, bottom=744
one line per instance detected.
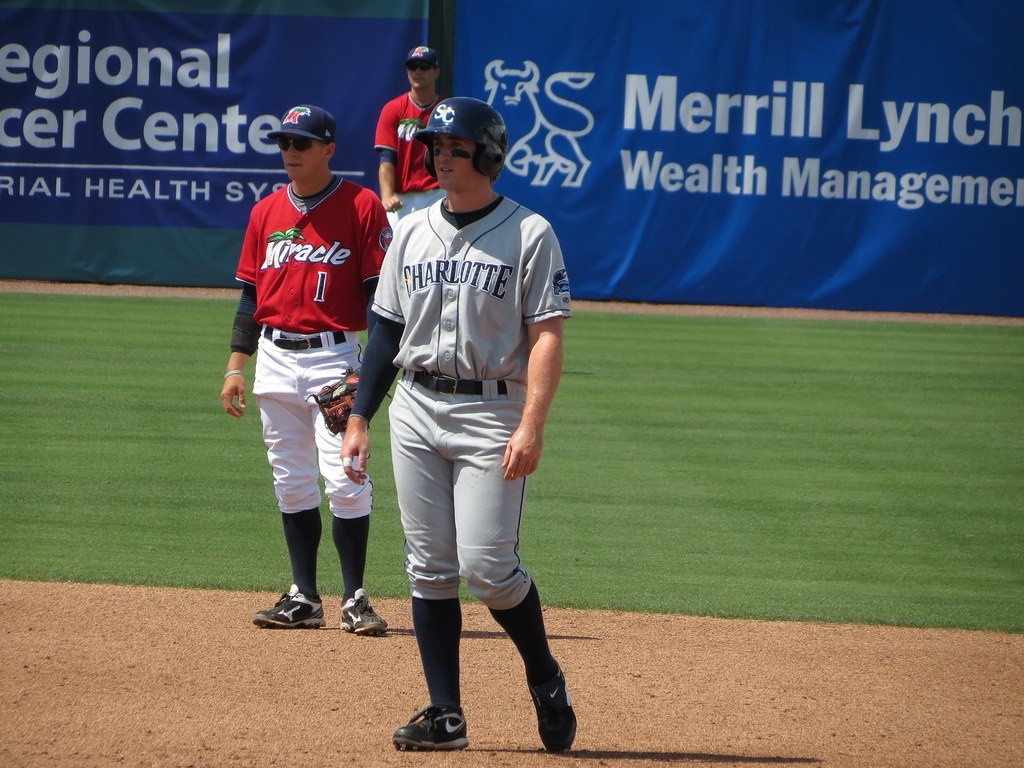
left=404, top=46, right=439, bottom=69
left=267, top=104, right=336, bottom=143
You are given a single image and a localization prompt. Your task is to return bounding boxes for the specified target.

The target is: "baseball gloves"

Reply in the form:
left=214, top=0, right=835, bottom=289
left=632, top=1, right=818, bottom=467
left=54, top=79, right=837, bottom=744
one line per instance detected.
left=312, top=366, right=361, bottom=434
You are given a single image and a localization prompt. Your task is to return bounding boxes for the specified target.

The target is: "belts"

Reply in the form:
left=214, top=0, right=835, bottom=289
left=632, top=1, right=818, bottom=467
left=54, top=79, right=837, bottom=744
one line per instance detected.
left=264, top=326, right=346, bottom=352
left=403, top=369, right=507, bottom=396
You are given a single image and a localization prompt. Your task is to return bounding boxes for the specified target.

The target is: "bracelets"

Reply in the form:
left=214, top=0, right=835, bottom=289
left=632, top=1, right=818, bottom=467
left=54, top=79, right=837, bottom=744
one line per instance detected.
left=224, top=369, right=241, bottom=380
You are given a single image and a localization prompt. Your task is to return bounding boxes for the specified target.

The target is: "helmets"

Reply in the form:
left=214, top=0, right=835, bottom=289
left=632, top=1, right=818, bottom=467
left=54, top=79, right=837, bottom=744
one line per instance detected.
left=412, top=96, right=508, bottom=181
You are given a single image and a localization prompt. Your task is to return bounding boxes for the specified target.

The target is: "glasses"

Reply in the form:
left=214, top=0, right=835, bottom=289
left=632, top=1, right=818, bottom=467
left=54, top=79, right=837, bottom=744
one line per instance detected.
left=406, top=60, right=434, bottom=71
left=275, top=134, right=329, bottom=152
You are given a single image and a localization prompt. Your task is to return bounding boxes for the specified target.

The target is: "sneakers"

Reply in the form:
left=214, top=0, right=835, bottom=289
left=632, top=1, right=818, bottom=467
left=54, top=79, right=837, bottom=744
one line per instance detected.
left=393, top=704, right=469, bottom=751
left=340, top=588, right=388, bottom=635
left=253, top=583, right=325, bottom=629
left=526, top=656, right=577, bottom=752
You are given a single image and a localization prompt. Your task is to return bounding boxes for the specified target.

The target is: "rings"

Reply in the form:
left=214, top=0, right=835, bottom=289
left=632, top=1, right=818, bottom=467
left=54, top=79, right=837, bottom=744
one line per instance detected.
left=343, top=457, right=353, bottom=467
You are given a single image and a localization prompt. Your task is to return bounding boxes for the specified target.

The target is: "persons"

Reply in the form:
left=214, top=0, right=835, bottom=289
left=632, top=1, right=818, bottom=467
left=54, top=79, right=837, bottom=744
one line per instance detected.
left=338, top=95, right=578, bottom=755
left=219, top=106, right=395, bottom=635
left=373, top=46, right=448, bottom=233
